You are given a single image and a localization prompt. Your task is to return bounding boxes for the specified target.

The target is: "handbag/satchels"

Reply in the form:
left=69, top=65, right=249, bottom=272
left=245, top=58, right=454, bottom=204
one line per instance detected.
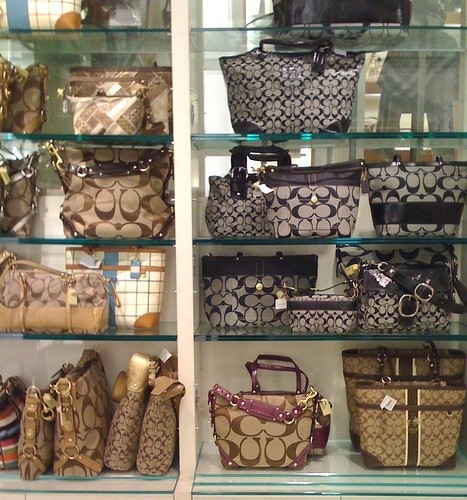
left=0, top=53, right=51, bottom=134
left=0, top=249, right=121, bottom=334
left=205, top=145, right=367, bottom=237
left=0, top=141, right=48, bottom=237
left=202, top=250, right=318, bottom=328
left=208, top=353, right=334, bottom=470
left=80, top=0, right=171, bottom=42
left=281, top=278, right=360, bottom=334
left=369, top=155, right=467, bottom=236
left=336, top=245, right=467, bottom=333
left=49, top=141, right=176, bottom=241
left=219, top=38, right=367, bottom=133
left=273, top=0, right=411, bottom=47
left=341, top=339, right=466, bottom=468
left=0, top=349, right=186, bottom=479
left=57, top=65, right=173, bottom=135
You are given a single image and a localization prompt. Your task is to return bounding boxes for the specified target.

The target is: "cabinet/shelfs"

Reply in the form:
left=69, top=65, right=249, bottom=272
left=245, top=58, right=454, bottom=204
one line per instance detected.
left=1, top=27, right=177, bottom=494
left=189, top=25, right=467, bottom=498
